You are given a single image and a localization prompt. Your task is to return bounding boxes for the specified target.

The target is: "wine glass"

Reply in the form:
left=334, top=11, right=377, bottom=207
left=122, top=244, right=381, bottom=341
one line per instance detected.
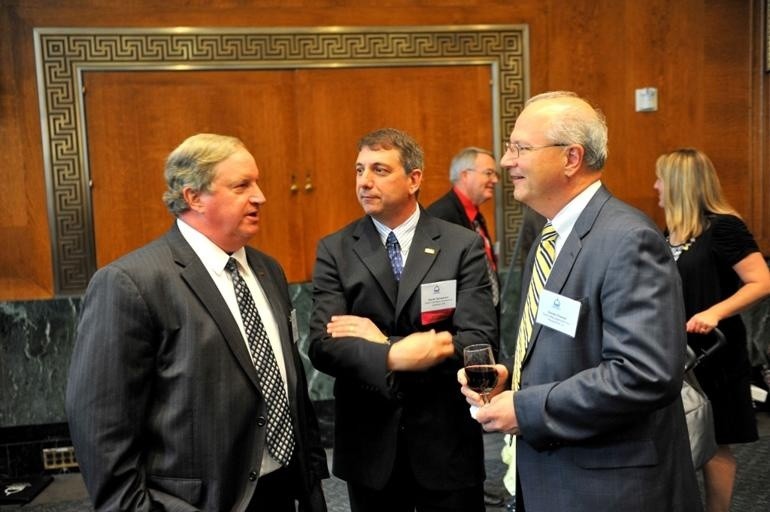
left=463, top=344, right=498, bottom=406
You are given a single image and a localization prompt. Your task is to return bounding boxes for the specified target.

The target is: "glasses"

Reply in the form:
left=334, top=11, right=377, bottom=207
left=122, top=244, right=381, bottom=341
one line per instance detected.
left=503, top=142, right=568, bottom=158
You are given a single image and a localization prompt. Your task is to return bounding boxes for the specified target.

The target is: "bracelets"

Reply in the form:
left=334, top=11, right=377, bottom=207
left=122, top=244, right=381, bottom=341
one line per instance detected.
left=383, top=335, right=390, bottom=345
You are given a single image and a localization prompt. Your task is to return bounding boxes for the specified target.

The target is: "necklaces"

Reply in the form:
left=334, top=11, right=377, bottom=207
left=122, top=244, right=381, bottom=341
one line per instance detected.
left=667, top=220, right=712, bottom=252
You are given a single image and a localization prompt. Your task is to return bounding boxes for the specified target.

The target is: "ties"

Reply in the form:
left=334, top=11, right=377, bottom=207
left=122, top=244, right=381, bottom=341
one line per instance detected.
left=508, top=222, right=558, bottom=447
left=386, top=232, right=403, bottom=288
left=224, top=257, right=296, bottom=468
left=473, top=220, right=500, bottom=307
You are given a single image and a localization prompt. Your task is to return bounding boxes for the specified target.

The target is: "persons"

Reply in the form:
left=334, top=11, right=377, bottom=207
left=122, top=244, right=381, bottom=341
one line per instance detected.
left=64, top=132, right=331, bottom=511
left=420, top=144, right=503, bottom=512
left=306, top=126, right=501, bottom=507
left=455, top=89, right=701, bottom=509
left=648, top=147, right=770, bottom=510
